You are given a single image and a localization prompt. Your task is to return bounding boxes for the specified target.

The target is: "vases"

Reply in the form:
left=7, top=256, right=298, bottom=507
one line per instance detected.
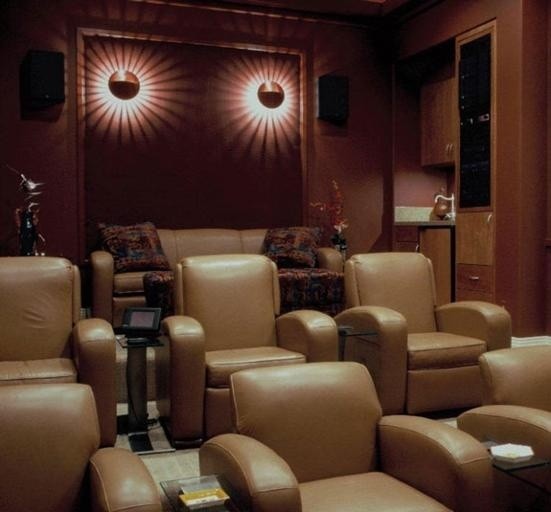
left=331, top=237, right=347, bottom=262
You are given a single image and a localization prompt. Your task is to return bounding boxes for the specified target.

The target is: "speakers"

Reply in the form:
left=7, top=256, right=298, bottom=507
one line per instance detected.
left=319, top=74, right=350, bottom=123
left=21, top=47, right=65, bottom=111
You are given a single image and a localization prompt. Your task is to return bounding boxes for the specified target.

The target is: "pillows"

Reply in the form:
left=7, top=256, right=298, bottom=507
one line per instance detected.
left=262, top=224, right=324, bottom=268
left=95, top=219, right=170, bottom=272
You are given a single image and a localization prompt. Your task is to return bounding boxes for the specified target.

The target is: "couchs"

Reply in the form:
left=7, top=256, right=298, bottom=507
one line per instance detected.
left=90, top=226, right=343, bottom=331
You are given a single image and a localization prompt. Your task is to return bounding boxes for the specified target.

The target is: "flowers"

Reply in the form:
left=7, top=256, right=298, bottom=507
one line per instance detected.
left=309, top=180, right=345, bottom=233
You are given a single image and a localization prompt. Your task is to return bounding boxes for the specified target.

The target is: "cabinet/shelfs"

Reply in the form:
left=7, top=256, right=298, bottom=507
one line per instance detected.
left=393, top=18, right=496, bottom=305
